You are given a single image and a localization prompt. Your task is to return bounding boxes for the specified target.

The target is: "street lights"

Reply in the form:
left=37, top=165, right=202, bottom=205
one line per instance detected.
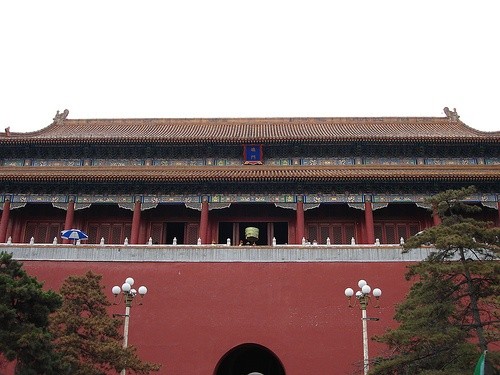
left=111, top=277, right=147, bottom=375
left=344, top=279, right=383, bottom=375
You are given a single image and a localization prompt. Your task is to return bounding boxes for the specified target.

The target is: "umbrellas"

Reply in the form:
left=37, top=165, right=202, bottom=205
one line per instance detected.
left=60, top=229, right=88, bottom=245
left=245, top=227, right=259, bottom=241
